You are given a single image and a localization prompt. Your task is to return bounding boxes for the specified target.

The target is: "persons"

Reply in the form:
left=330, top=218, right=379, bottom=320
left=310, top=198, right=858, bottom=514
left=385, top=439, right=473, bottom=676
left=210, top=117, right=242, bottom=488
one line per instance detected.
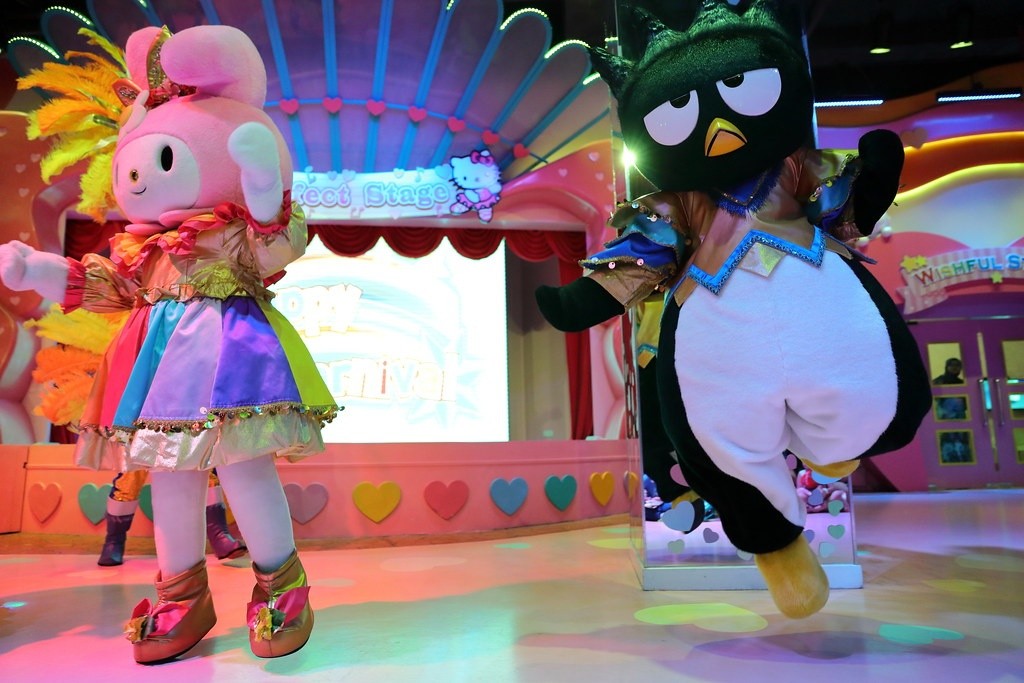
left=97, top=468, right=249, bottom=566
left=932, top=358, right=964, bottom=385
left=942, top=436, right=965, bottom=463
left=642, top=459, right=850, bottom=522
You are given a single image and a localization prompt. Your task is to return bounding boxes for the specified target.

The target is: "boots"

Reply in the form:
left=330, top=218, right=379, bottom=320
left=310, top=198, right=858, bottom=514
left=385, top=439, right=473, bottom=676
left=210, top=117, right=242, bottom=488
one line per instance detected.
left=98, top=496, right=138, bottom=566
left=204, top=486, right=247, bottom=560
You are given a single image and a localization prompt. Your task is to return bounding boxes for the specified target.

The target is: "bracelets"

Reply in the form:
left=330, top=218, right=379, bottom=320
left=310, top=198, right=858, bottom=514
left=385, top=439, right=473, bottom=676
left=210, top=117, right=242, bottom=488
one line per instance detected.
left=822, top=487, right=829, bottom=494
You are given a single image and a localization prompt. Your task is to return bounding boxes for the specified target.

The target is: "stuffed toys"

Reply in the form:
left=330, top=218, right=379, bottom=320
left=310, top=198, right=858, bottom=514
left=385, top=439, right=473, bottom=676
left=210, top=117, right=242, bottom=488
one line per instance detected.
left=0, top=25, right=345, bottom=666
left=536, top=0, right=932, bottom=620
left=637, top=292, right=708, bottom=534
